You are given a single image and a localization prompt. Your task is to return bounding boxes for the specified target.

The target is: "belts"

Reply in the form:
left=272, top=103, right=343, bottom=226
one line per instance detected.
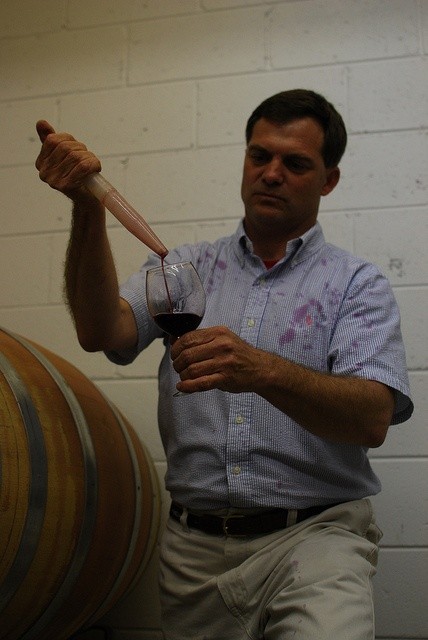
left=169, top=501, right=346, bottom=540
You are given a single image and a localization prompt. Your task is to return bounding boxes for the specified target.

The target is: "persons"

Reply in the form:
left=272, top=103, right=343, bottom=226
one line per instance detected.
left=36, top=90, right=414, bottom=638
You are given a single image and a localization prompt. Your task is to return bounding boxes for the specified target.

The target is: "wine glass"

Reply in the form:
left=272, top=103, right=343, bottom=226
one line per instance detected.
left=146, top=261, right=205, bottom=396
